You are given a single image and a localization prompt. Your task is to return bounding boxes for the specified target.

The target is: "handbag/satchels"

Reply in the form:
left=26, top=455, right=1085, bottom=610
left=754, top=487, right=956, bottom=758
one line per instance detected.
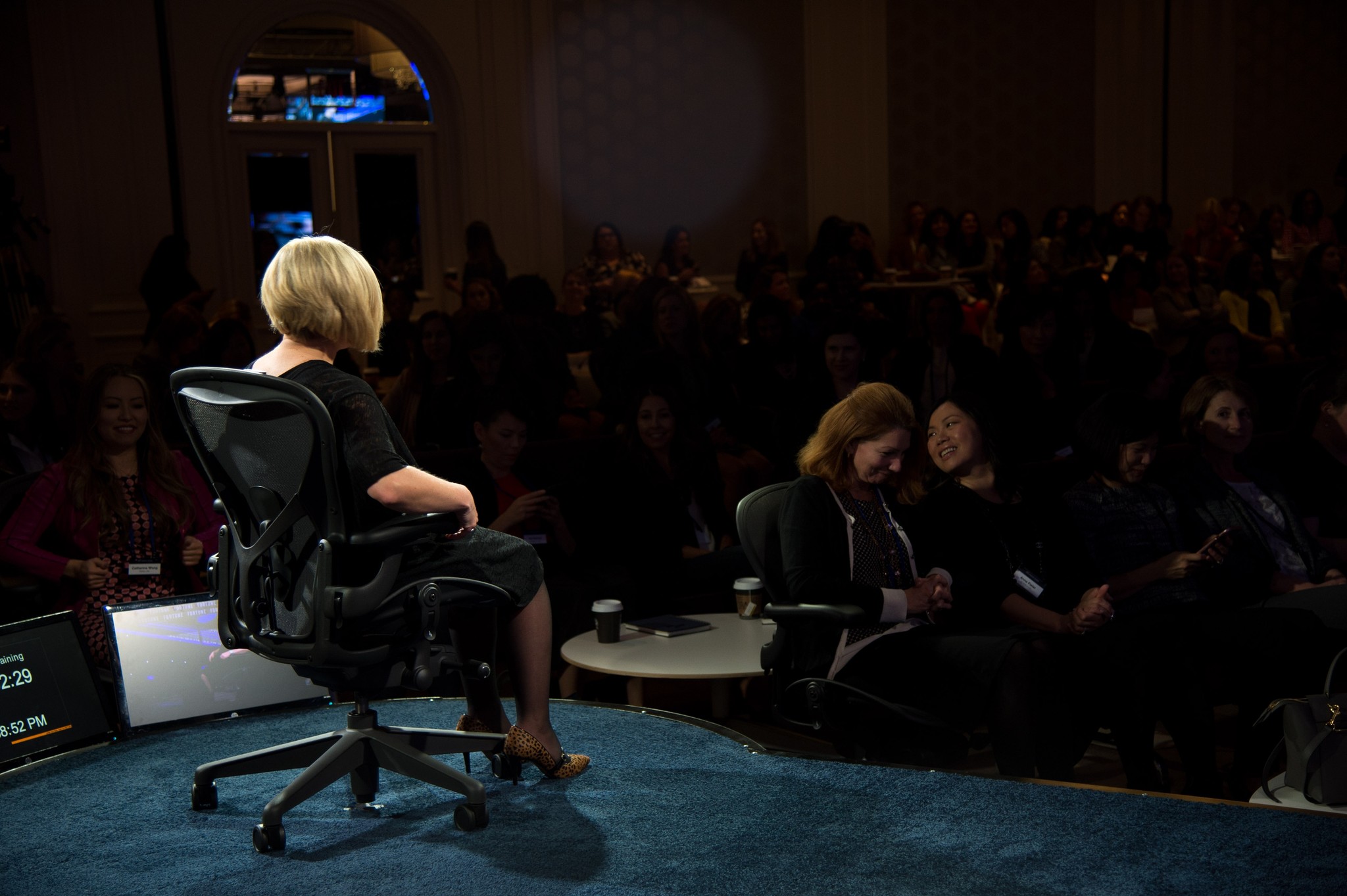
left=1252, top=646, right=1347, bottom=809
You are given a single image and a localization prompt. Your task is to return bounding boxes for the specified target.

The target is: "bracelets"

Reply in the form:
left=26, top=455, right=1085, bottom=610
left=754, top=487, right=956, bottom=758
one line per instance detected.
left=1109, top=611, right=1115, bottom=621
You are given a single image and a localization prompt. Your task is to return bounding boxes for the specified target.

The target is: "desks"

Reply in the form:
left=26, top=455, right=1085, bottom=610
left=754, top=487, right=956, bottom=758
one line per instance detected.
left=561, top=611, right=790, bottom=731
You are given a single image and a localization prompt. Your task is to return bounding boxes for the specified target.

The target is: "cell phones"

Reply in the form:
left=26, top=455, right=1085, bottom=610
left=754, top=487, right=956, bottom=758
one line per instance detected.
left=1196, top=526, right=1244, bottom=555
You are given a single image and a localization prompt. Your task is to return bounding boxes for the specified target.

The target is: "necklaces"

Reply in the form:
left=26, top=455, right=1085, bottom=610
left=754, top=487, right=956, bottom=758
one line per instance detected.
left=491, top=476, right=518, bottom=500
left=107, top=457, right=137, bottom=476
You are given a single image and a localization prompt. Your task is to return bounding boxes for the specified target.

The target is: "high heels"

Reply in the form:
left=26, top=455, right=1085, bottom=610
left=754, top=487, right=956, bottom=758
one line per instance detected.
left=456, top=714, right=527, bottom=774
left=503, top=725, right=590, bottom=786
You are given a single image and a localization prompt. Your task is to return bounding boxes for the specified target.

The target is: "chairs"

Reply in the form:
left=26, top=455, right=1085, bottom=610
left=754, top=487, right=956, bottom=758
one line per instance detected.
left=171, top=367, right=520, bottom=863
left=744, top=481, right=901, bottom=764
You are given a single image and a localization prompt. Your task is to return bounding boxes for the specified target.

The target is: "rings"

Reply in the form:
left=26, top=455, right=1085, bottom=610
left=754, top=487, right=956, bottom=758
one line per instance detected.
left=1216, top=557, right=1223, bottom=564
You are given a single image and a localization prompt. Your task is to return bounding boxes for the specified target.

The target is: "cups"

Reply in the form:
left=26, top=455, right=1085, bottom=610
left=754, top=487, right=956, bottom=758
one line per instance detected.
left=591, top=600, right=623, bottom=644
left=733, top=577, right=762, bottom=619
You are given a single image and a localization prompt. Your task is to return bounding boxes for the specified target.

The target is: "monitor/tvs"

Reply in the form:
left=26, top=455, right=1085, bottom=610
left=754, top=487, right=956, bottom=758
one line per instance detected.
left=102, top=588, right=330, bottom=744
left=0, top=609, right=116, bottom=782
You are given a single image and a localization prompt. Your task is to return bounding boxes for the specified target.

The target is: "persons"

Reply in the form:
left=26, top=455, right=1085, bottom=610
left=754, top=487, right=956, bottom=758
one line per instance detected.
left=0, top=366, right=231, bottom=684
left=451, top=393, right=597, bottom=701
left=229, top=230, right=594, bottom=787
left=364, top=186, right=1347, bottom=474
left=598, top=388, right=761, bottom=615
left=0, top=223, right=259, bottom=577
left=1034, top=400, right=1251, bottom=799
left=1290, top=388, right=1347, bottom=566
left=789, top=379, right=1054, bottom=779
left=1169, top=376, right=1346, bottom=685
left=907, top=393, right=1123, bottom=781
